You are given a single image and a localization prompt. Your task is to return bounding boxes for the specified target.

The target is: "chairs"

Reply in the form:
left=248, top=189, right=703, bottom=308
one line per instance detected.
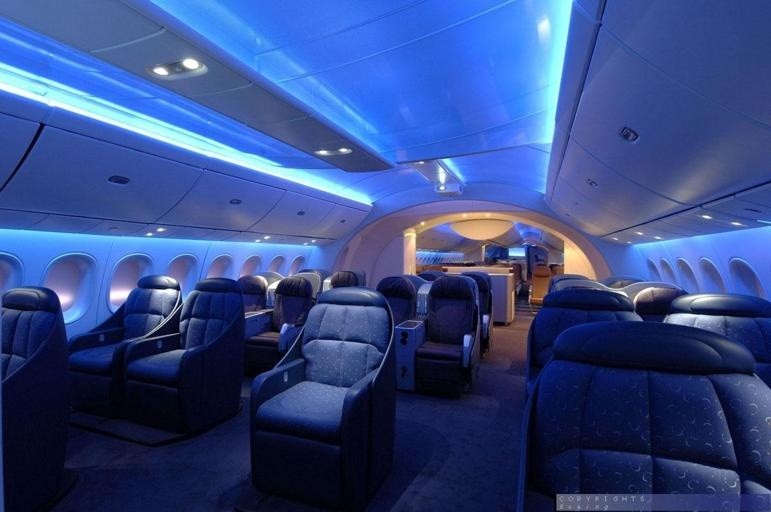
left=0, top=262, right=771, bottom=511
